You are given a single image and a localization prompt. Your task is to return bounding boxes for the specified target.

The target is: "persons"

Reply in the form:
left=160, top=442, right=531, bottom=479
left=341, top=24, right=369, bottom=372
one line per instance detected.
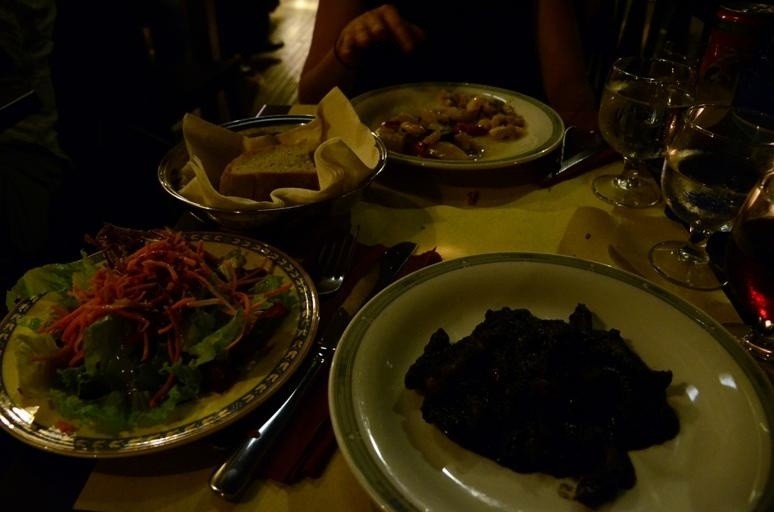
left=0, top=0, right=62, bottom=254
left=298, top=0, right=596, bottom=129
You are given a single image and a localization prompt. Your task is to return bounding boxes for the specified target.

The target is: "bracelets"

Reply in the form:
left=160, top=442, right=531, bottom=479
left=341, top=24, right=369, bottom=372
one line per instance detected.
left=333, top=33, right=348, bottom=65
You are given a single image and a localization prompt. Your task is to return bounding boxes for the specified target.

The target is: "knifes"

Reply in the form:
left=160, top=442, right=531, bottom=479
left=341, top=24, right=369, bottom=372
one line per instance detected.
left=211, top=240, right=417, bottom=500
left=545, top=135, right=607, bottom=179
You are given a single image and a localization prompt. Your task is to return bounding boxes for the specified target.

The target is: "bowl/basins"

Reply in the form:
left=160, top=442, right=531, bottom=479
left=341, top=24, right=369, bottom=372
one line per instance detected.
left=156, top=114, right=389, bottom=230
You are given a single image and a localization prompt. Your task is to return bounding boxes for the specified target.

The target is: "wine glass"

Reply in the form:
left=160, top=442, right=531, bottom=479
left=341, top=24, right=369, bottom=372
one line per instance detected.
left=649, top=97, right=774, bottom=289
left=591, top=54, right=702, bottom=210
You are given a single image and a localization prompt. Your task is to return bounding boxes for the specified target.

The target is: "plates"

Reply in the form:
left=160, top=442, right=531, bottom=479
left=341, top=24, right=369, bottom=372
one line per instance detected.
left=351, top=82, right=564, bottom=171
left=4, top=229, right=321, bottom=457
left=329, top=250, right=771, bottom=510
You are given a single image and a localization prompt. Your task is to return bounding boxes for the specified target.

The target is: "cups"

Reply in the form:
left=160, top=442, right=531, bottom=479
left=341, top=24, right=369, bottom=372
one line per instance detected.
left=723, top=167, right=774, bottom=358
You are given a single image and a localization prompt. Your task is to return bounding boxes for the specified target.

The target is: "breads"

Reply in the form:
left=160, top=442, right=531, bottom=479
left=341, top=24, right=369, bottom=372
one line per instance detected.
left=218, top=142, right=320, bottom=202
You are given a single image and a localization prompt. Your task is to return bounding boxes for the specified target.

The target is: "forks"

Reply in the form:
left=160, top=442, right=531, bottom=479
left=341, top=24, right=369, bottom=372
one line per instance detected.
left=310, top=234, right=355, bottom=296
